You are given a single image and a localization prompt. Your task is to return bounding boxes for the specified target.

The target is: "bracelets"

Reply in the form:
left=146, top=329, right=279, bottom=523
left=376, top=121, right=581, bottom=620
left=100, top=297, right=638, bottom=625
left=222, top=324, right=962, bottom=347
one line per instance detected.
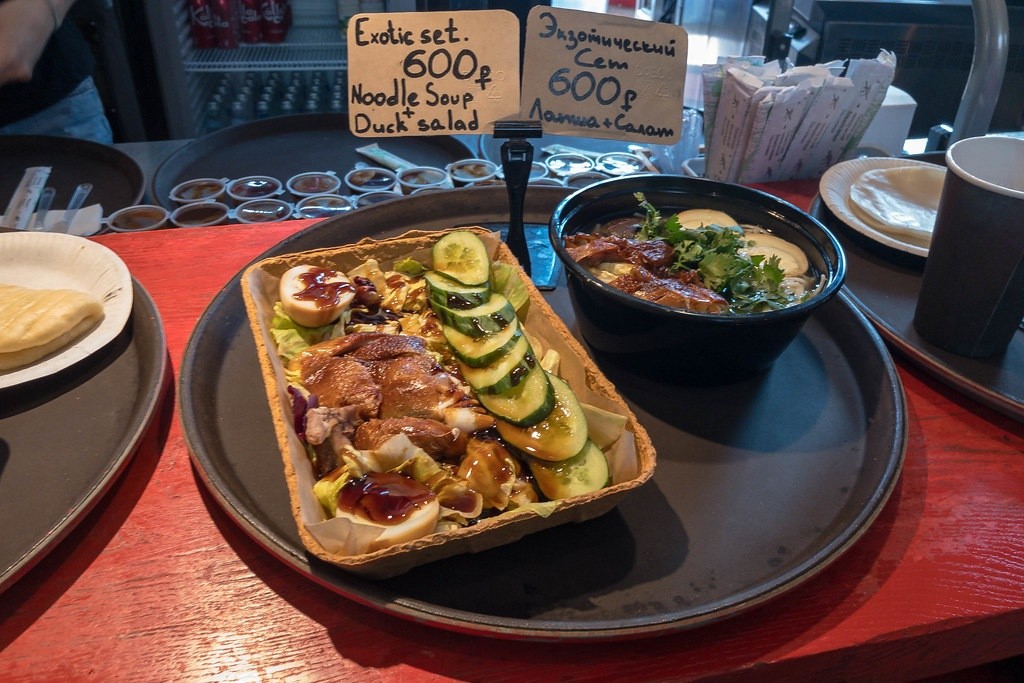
left=49, top=1, right=62, bottom=31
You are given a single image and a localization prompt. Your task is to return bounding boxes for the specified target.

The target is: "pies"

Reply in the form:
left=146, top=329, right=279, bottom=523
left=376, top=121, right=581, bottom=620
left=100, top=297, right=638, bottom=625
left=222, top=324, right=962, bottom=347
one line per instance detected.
left=849, top=165, right=948, bottom=241
left=0, top=283, right=105, bottom=372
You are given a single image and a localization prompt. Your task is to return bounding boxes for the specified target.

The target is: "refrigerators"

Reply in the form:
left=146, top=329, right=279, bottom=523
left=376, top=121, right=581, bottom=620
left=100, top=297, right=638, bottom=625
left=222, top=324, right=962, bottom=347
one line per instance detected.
left=145, top=0, right=431, bottom=141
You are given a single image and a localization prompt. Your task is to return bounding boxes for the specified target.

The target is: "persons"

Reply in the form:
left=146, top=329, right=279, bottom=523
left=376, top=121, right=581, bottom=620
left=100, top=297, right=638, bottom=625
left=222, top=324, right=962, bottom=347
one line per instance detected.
left=0, top=1, right=114, bottom=145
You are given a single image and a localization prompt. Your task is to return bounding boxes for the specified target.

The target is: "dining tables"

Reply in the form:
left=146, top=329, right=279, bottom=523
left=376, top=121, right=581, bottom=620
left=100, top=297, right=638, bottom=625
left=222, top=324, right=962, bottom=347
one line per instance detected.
left=0, top=170, right=1024, bottom=683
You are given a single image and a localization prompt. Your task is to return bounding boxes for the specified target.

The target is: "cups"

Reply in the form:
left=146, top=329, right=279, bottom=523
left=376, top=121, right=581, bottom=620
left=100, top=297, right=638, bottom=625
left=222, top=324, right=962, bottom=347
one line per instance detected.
left=914, top=137, right=1024, bottom=364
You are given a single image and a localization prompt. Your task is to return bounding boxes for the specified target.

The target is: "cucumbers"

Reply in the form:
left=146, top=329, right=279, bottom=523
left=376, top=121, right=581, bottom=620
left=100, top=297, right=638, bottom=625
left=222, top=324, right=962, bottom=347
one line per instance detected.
left=422, top=230, right=610, bottom=504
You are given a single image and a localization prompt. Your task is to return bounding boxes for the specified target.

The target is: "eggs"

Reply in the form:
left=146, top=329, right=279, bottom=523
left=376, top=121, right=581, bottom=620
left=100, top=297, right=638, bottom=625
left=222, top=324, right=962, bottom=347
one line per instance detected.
left=335, top=480, right=441, bottom=549
left=279, top=264, right=355, bottom=327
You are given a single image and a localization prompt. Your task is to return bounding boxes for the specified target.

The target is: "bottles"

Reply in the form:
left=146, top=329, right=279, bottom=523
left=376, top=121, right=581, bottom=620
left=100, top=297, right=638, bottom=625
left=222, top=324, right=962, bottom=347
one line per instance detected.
left=203, top=72, right=349, bottom=140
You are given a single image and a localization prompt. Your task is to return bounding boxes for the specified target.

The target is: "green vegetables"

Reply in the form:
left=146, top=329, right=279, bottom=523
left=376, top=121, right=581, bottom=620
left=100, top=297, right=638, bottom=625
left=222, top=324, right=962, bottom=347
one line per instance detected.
left=631, top=191, right=811, bottom=316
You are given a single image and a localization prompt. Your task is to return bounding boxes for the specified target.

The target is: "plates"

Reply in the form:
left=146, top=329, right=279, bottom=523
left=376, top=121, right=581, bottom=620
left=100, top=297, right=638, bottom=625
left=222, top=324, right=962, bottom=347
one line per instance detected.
left=0, top=231, right=134, bottom=391
left=817, top=157, right=954, bottom=261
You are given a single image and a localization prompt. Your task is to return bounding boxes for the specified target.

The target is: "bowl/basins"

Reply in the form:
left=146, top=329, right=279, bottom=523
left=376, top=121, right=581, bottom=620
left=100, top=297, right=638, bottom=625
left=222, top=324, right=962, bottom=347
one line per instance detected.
left=547, top=174, right=847, bottom=384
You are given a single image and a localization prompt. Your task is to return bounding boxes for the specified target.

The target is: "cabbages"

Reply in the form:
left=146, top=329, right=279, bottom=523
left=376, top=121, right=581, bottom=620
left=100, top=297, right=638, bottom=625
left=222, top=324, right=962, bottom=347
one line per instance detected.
left=270, top=255, right=539, bottom=533
